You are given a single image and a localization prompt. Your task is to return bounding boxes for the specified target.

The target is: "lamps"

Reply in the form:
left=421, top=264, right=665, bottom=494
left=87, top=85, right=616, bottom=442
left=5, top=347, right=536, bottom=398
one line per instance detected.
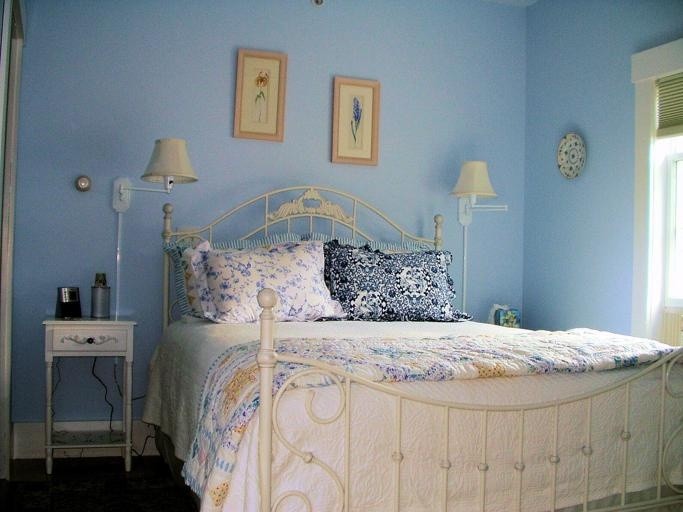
left=448, top=159, right=508, bottom=313
left=110, top=137, right=198, bottom=322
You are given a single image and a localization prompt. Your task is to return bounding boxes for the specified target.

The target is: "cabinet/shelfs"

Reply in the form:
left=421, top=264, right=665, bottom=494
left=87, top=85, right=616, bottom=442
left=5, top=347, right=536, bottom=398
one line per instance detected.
left=41, top=318, right=139, bottom=475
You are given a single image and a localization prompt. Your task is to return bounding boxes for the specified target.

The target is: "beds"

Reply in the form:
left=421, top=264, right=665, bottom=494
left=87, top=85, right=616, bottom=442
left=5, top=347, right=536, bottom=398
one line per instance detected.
left=162, top=185, right=682, bottom=512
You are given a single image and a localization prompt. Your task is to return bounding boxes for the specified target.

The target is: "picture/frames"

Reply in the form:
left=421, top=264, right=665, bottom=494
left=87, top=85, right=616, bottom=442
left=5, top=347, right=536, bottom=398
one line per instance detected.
left=231, top=45, right=286, bottom=144
left=329, top=72, right=379, bottom=167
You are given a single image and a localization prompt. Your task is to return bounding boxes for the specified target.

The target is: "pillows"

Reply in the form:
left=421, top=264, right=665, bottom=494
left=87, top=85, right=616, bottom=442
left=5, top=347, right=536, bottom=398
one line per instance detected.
left=164, top=229, right=304, bottom=323
left=323, top=239, right=473, bottom=324
left=186, top=241, right=345, bottom=323
left=304, top=228, right=430, bottom=251
